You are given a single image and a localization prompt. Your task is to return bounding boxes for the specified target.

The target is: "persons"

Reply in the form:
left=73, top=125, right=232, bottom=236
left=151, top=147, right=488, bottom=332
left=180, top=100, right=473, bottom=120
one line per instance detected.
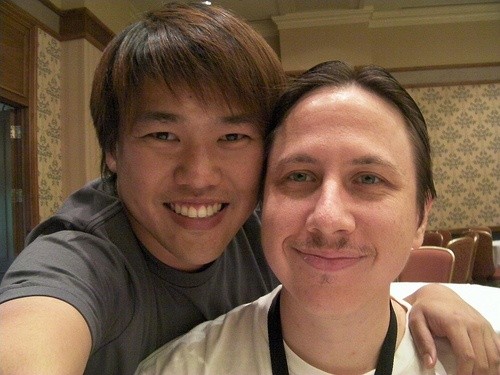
left=131, top=60, right=499, bottom=375
left=2, top=3, right=499, bottom=375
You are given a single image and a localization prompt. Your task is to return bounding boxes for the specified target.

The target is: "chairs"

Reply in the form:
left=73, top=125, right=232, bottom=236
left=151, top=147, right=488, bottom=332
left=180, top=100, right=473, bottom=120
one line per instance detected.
left=397, top=227, right=500, bottom=285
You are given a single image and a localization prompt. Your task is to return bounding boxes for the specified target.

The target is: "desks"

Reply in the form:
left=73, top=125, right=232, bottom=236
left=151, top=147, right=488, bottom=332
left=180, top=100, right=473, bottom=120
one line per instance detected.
left=390, top=281, right=500, bottom=334
